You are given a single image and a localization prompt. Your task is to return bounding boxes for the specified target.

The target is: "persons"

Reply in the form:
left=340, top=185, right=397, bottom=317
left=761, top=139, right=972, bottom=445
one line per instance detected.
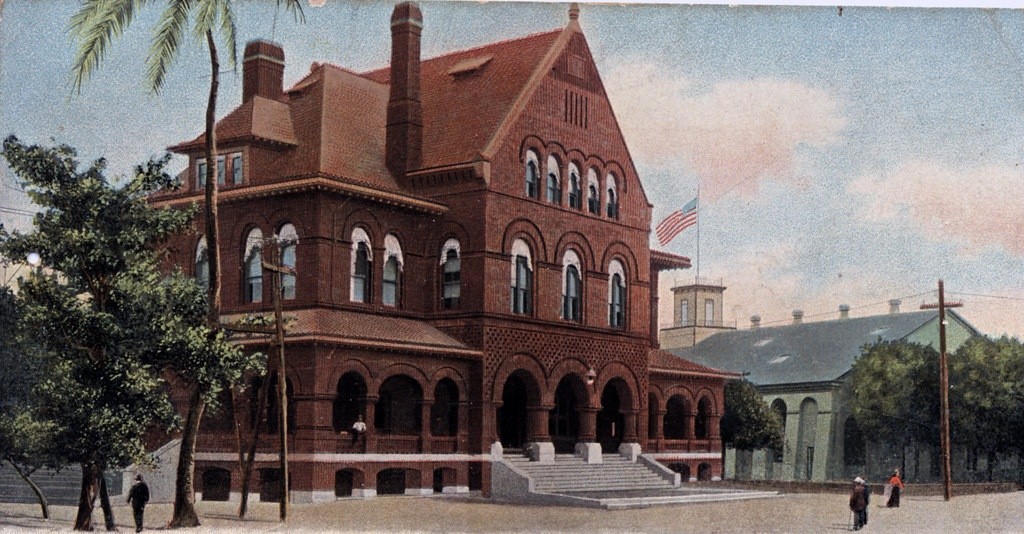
left=886, top=468, right=904, bottom=508
left=127, top=474, right=150, bottom=533
left=850, top=476, right=866, bottom=531
left=859, top=479, right=869, bottom=525
left=352, top=414, right=367, bottom=431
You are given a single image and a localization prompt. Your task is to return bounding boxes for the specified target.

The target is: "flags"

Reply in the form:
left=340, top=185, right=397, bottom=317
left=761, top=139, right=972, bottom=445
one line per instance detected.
left=655, top=196, right=697, bottom=247
